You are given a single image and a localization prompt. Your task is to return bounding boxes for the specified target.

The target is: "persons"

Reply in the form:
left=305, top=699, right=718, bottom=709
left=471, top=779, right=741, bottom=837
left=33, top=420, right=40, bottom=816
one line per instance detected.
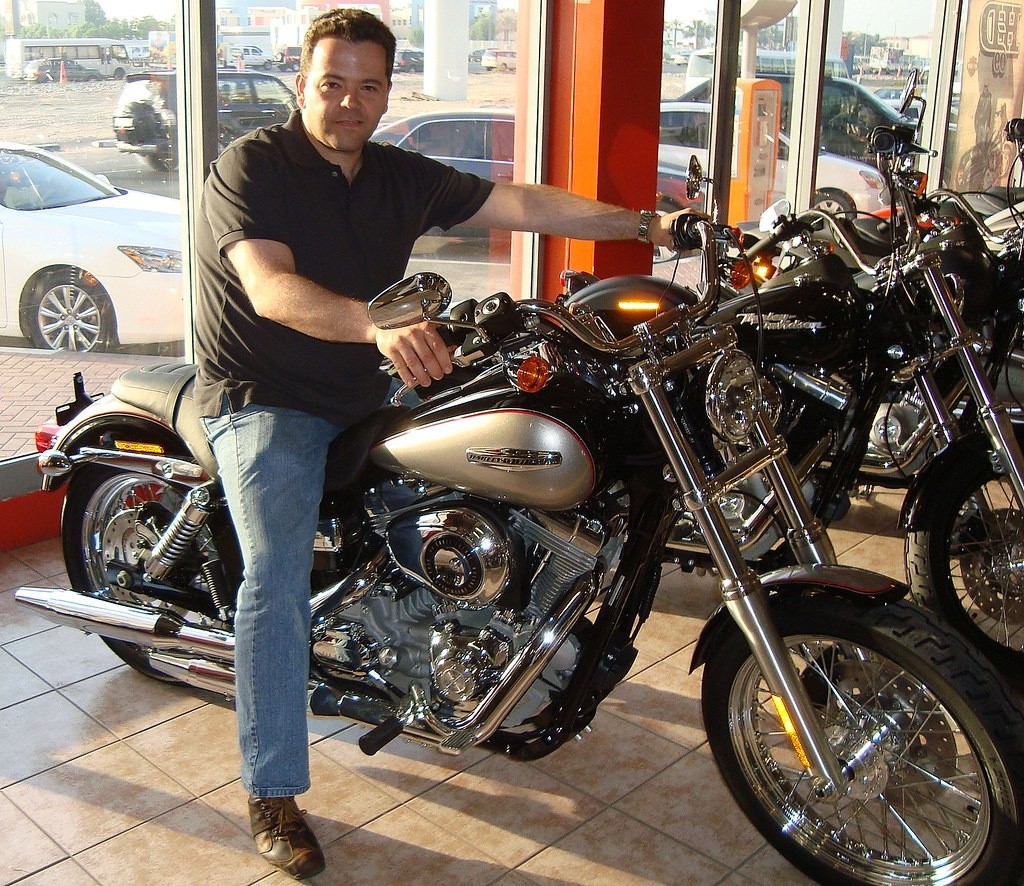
left=194, top=8, right=712, bottom=880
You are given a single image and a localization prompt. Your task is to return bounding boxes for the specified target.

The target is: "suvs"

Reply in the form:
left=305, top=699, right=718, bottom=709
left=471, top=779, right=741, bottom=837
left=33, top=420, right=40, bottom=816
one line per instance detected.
left=393, top=50, right=424, bottom=74
left=27, top=58, right=104, bottom=83
left=110, top=69, right=298, bottom=170
left=277, top=45, right=301, bottom=71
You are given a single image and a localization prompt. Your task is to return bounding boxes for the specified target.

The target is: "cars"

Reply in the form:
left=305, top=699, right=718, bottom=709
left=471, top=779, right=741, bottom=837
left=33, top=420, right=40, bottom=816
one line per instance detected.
left=367, top=109, right=515, bottom=243
left=653, top=74, right=918, bottom=263
left=224, top=44, right=273, bottom=69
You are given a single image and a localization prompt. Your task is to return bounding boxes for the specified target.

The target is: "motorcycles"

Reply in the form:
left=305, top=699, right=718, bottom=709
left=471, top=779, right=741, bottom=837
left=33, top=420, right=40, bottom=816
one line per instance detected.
left=15, top=66, right=1024, bottom=886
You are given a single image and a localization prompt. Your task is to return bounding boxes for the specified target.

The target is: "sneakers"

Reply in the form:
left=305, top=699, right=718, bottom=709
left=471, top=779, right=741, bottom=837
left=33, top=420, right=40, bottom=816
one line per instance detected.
left=248, top=794, right=325, bottom=880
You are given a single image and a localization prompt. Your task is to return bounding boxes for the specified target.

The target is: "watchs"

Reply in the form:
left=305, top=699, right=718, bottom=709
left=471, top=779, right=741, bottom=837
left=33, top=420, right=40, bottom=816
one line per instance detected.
left=637, top=209, right=661, bottom=243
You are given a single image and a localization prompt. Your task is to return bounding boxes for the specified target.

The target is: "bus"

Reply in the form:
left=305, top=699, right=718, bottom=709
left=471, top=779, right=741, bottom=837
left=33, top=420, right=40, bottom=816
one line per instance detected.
left=2, top=38, right=131, bottom=82
left=680, top=47, right=850, bottom=92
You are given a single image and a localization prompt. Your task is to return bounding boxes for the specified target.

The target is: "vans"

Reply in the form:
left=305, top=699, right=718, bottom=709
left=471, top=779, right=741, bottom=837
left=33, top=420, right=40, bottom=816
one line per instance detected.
left=480, top=47, right=517, bottom=71
